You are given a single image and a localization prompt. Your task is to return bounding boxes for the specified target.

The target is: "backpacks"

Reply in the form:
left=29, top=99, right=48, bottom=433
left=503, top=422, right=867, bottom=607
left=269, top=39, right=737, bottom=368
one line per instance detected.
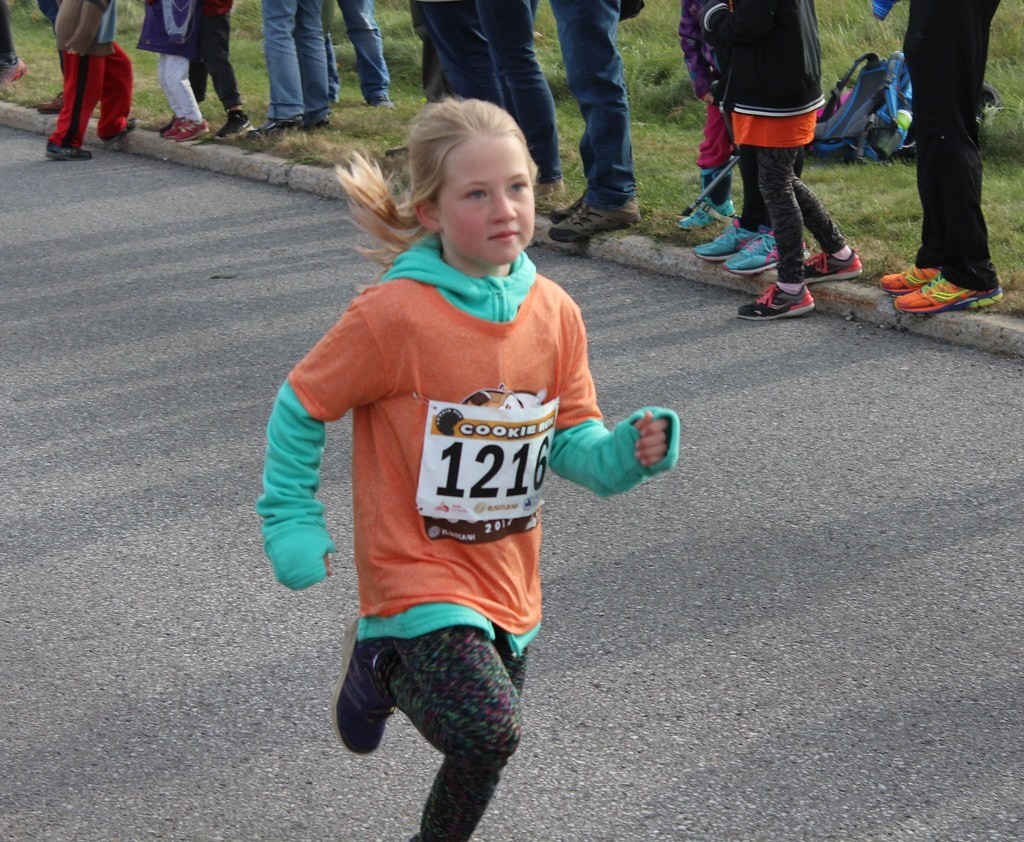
left=813, top=51, right=913, bottom=163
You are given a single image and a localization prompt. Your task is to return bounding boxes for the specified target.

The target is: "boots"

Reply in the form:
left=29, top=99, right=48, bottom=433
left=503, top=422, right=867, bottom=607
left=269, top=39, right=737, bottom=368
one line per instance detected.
left=676, top=167, right=736, bottom=229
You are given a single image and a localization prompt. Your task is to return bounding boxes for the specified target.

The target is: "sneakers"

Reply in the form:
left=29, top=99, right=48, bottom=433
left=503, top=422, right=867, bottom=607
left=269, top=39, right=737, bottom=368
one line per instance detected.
left=738, top=283, right=814, bottom=320
left=102, top=117, right=137, bottom=144
left=246, top=116, right=303, bottom=138
left=174, top=119, right=210, bottom=141
left=723, top=225, right=806, bottom=275
left=160, top=119, right=173, bottom=133
left=894, top=272, right=1003, bottom=316
left=693, top=219, right=761, bottom=260
left=214, top=107, right=251, bottom=139
left=803, top=249, right=862, bottom=285
left=0, top=60, right=27, bottom=86
left=880, top=267, right=941, bottom=295
left=548, top=195, right=640, bottom=242
left=331, top=620, right=395, bottom=753
left=303, top=118, right=329, bottom=130
left=38, top=90, right=63, bottom=112
left=46, top=141, right=91, bottom=160
left=162, top=118, right=184, bottom=138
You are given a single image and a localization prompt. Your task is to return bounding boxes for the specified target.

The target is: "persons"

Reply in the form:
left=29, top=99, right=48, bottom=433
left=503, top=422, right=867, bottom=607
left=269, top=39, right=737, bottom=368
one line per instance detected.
left=136, top=1, right=256, bottom=141
left=322, top=0, right=394, bottom=110
left=700, top=0, right=864, bottom=321
left=408, top=0, right=566, bottom=200
left=0, top=0, right=27, bottom=84
left=545, top=0, right=640, bottom=243
left=243, top=0, right=332, bottom=138
left=35, top=0, right=139, bottom=160
left=675, top=0, right=812, bottom=277
left=256, top=98, right=680, bottom=842
left=866, top=0, right=1004, bottom=316
